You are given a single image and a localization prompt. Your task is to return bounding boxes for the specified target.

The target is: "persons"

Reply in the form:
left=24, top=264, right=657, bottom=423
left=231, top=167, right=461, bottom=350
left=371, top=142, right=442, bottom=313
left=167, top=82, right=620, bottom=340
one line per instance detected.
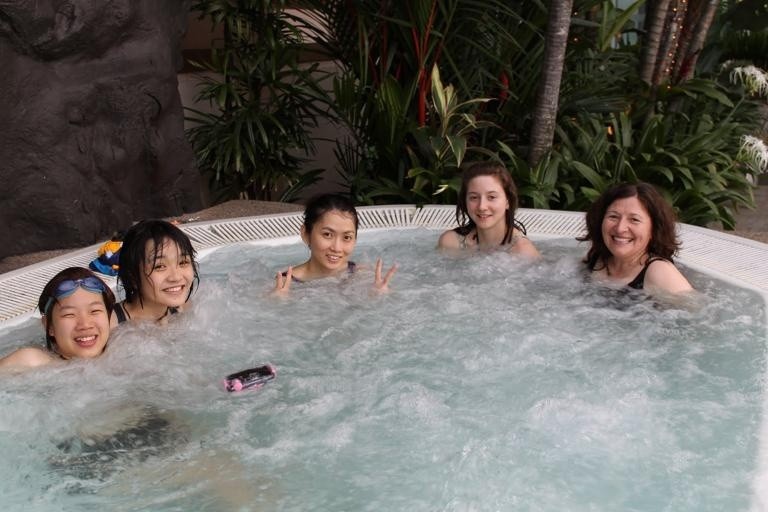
left=271, top=191, right=397, bottom=293
left=0, top=269, right=115, bottom=375
left=438, top=161, right=540, bottom=259
left=110, top=219, right=200, bottom=332
left=575, top=180, right=696, bottom=301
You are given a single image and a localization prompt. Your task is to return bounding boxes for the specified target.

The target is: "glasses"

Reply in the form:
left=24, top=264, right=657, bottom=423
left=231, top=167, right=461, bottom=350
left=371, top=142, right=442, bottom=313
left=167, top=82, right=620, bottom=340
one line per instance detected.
left=45, top=277, right=103, bottom=315
